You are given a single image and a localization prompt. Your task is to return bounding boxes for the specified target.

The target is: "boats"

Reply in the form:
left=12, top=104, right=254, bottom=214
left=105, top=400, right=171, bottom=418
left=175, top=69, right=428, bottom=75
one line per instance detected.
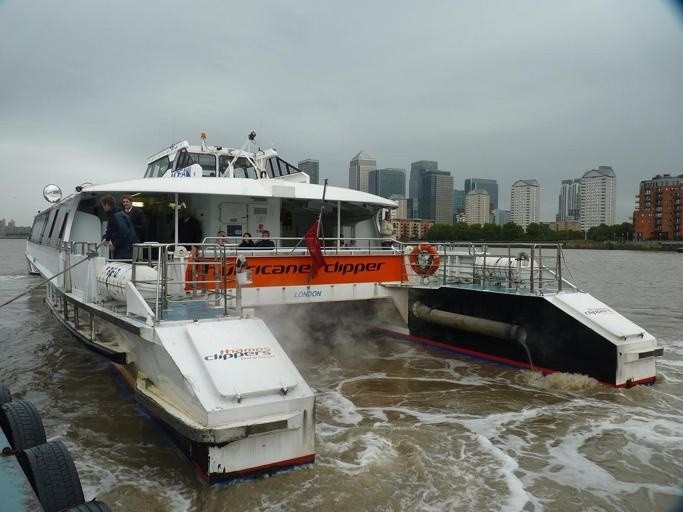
left=19, top=127, right=664, bottom=488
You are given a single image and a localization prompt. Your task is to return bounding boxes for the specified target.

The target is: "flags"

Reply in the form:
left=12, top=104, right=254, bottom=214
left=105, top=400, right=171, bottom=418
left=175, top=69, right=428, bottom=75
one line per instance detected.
left=302, top=218, right=326, bottom=269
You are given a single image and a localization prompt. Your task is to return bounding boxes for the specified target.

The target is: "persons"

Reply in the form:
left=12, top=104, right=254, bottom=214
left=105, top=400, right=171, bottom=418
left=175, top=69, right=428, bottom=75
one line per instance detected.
left=178, top=207, right=204, bottom=251
left=236, top=232, right=255, bottom=250
left=99, top=193, right=142, bottom=264
left=255, top=230, right=275, bottom=251
left=333, top=232, right=346, bottom=250
left=346, top=237, right=360, bottom=250
left=215, top=230, right=231, bottom=250
left=378, top=210, right=397, bottom=250
left=119, top=195, right=147, bottom=247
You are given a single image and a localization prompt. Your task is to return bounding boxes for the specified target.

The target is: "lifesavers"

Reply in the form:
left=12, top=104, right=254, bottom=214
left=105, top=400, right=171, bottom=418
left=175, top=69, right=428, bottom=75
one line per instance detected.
left=409, top=244, right=439, bottom=278
